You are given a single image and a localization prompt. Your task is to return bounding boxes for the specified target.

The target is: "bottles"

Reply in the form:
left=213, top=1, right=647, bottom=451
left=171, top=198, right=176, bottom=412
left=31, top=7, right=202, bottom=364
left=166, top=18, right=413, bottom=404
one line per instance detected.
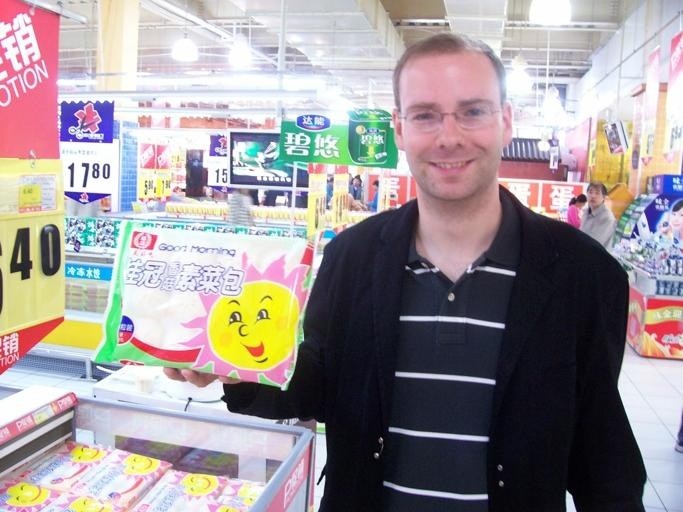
left=662, top=209, right=670, bottom=233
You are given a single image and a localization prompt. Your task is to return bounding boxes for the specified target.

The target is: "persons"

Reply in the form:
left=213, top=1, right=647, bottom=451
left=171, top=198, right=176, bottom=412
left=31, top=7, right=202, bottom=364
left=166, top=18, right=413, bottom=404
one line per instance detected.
left=171, top=184, right=182, bottom=202
left=326, top=172, right=378, bottom=213
left=162, top=34, right=648, bottom=511
left=578, top=180, right=616, bottom=254
left=657, top=199, right=682, bottom=255
left=566, top=192, right=587, bottom=229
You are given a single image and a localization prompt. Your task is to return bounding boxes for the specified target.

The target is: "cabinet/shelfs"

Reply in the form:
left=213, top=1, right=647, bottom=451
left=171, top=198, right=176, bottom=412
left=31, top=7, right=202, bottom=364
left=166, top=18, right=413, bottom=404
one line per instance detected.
left=0, top=385, right=315, bottom=512
left=612, top=251, right=683, bottom=360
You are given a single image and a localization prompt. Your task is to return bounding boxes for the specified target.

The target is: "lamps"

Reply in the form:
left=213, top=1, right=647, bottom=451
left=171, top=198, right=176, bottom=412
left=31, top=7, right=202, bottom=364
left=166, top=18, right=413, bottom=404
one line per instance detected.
left=229, top=0, right=254, bottom=66
left=170, top=1, right=199, bottom=64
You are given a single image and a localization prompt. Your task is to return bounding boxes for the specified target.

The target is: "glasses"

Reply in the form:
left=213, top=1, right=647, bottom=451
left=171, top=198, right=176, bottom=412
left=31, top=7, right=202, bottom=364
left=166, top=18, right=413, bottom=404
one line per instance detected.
left=397, top=107, right=503, bottom=133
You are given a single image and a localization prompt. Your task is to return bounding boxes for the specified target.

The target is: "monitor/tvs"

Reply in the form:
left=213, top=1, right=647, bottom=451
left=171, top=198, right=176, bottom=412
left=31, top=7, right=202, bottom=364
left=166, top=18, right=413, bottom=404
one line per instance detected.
left=227, top=128, right=308, bottom=191
left=602, top=121, right=629, bottom=154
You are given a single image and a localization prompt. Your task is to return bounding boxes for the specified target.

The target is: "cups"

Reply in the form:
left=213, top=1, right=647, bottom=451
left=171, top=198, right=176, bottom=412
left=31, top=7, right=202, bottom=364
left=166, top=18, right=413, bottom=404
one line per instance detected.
left=346, top=108, right=392, bottom=166
left=656, top=255, right=682, bottom=296
